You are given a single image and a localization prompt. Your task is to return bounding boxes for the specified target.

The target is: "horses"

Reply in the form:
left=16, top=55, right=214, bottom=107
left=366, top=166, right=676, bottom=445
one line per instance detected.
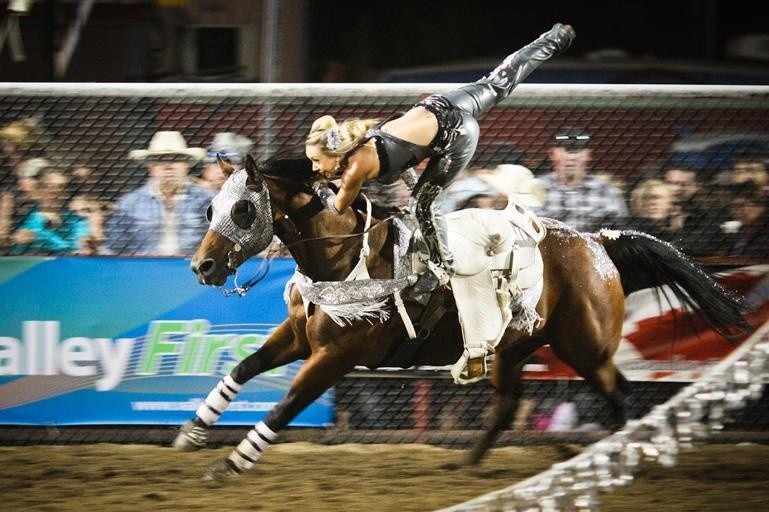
left=172, top=153, right=755, bottom=489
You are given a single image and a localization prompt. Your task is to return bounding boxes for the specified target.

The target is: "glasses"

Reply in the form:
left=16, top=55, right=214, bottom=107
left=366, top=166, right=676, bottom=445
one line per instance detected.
left=551, top=133, right=592, bottom=142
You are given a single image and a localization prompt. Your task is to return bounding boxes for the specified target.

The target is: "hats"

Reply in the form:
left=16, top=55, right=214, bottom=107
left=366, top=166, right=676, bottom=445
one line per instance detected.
left=16, top=156, right=57, bottom=180
left=128, top=129, right=208, bottom=169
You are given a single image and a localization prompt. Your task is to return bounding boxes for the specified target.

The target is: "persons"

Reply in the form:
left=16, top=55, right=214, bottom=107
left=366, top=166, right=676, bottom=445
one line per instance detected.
left=0, top=115, right=296, bottom=256
left=333, top=114, right=768, bottom=428
left=304, top=22, right=576, bottom=301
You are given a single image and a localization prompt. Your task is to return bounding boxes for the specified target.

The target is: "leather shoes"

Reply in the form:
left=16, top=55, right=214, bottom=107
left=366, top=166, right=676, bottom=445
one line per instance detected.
left=412, top=269, right=454, bottom=294
left=552, top=22, right=577, bottom=40
